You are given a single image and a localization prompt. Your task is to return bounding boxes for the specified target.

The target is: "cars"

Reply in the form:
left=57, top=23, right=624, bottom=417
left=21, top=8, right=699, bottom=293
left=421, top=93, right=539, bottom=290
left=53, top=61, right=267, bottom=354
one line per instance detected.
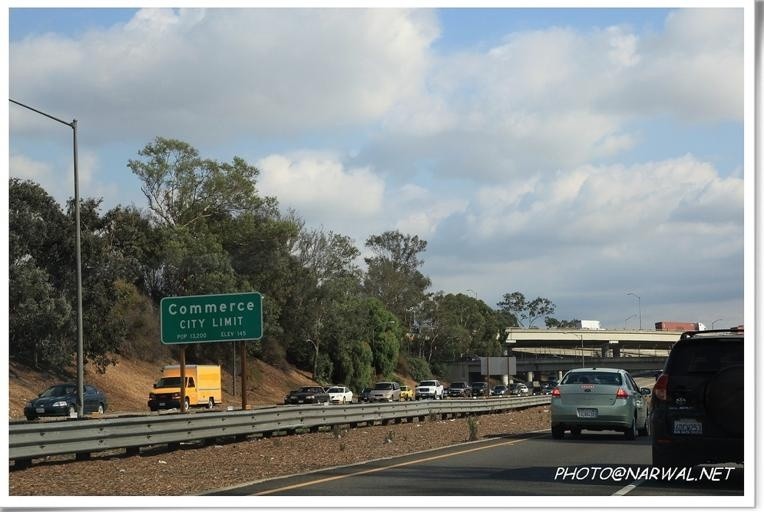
left=283, top=379, right=557, bottom=404
left=550, top=367, right=650, bottom=440
left=23, top=383, right=107, bottom=421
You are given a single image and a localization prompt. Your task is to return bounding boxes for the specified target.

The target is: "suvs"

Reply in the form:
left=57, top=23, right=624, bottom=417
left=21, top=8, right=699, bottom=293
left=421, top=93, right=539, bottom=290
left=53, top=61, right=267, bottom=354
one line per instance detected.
left=648, top=328, right=744, bottom=481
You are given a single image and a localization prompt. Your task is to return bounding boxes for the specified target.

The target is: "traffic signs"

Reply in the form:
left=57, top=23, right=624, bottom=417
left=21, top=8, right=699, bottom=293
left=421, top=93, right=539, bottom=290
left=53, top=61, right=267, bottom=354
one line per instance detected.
left=159, top=291, right=263, bottom=345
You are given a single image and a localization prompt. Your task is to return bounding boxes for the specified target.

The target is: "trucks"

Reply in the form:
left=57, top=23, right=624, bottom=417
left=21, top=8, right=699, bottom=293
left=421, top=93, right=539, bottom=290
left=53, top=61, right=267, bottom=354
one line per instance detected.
left=147, top=363, right=221, bottom=412
